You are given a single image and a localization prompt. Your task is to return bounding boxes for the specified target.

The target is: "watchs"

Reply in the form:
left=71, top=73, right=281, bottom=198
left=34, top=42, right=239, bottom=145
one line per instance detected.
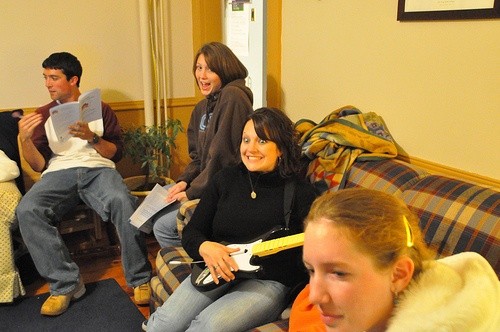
left=88, top=132, right=99, bottom=146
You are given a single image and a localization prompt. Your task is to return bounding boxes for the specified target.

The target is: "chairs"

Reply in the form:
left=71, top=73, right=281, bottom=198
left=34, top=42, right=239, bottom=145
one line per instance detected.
left=0, top=109, right=30, bottom=302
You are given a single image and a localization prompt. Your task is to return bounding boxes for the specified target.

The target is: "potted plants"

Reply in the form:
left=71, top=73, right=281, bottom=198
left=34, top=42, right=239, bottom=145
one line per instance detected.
left=119, top=117, right=187, bottom=209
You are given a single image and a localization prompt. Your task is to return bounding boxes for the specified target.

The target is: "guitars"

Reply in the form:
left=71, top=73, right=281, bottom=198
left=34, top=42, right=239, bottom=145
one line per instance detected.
left=190, top=232, right=305, bottom=293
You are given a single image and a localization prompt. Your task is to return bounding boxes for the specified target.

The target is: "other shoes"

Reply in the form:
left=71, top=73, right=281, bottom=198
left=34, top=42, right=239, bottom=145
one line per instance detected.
left=133, top=280, right=153, bottom=305
left=40, top=272, right=86, bottom=316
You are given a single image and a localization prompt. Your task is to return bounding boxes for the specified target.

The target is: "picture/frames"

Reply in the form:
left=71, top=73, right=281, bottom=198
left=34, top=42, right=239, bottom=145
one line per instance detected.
left=396, top=0, right=500, bottom=22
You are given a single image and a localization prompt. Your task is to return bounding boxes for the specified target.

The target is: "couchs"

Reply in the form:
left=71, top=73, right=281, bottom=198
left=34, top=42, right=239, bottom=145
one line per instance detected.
left=150, top=120, right=500, bottom=332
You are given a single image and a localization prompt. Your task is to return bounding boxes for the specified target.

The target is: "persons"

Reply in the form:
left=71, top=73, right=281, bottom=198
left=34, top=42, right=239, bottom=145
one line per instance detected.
left=288, top=188, right=500, bottom=332
left=15, top=52, right=151, bottom=315
left=142, top=107, right=322, bottom=332
left=137, top=41, right=254, bottom=248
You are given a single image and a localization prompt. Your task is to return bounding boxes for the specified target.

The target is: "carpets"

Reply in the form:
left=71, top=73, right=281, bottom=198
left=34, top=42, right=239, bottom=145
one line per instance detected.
left=0, top=277, right=149, bottom=332
